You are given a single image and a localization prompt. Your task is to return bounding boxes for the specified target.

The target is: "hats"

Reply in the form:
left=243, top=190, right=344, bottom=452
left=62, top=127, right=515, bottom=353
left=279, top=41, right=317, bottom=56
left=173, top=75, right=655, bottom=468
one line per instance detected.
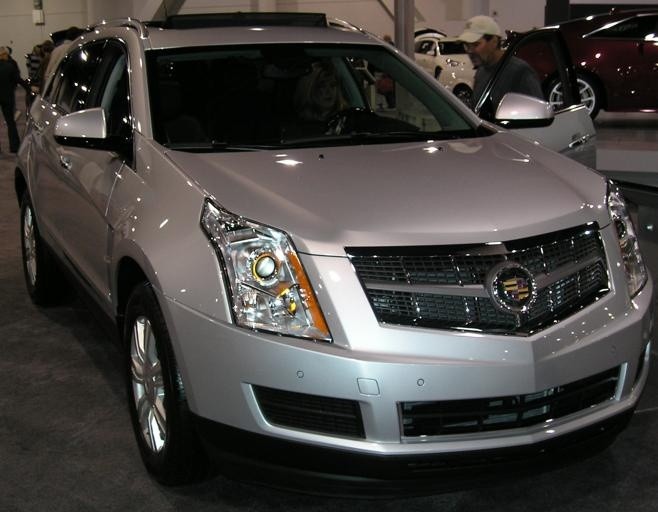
left=454, top=14, right=502, bottom=44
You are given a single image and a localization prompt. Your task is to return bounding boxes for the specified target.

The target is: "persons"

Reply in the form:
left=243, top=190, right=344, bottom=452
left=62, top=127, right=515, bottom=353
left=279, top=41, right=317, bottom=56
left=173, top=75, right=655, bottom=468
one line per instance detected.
left=0, top=27, right=80, bottom=153
left=292, top=62, right=350, bottom=140
left=456, top=15, right=545, bottom=110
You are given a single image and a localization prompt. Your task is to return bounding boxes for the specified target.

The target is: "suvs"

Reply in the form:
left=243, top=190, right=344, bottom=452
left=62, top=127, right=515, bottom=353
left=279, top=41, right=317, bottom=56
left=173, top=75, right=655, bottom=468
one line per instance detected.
left=10, top=8, right=654, bottom=482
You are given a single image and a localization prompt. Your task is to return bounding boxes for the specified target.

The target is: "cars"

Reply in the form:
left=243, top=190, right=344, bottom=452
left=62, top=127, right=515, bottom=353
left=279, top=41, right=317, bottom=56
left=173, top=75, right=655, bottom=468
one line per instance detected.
left=411, top=25, right=476, bottom=82
left=436, top=38, right=511, bottom=113
left=505, top=6, right=658, bottom=126
